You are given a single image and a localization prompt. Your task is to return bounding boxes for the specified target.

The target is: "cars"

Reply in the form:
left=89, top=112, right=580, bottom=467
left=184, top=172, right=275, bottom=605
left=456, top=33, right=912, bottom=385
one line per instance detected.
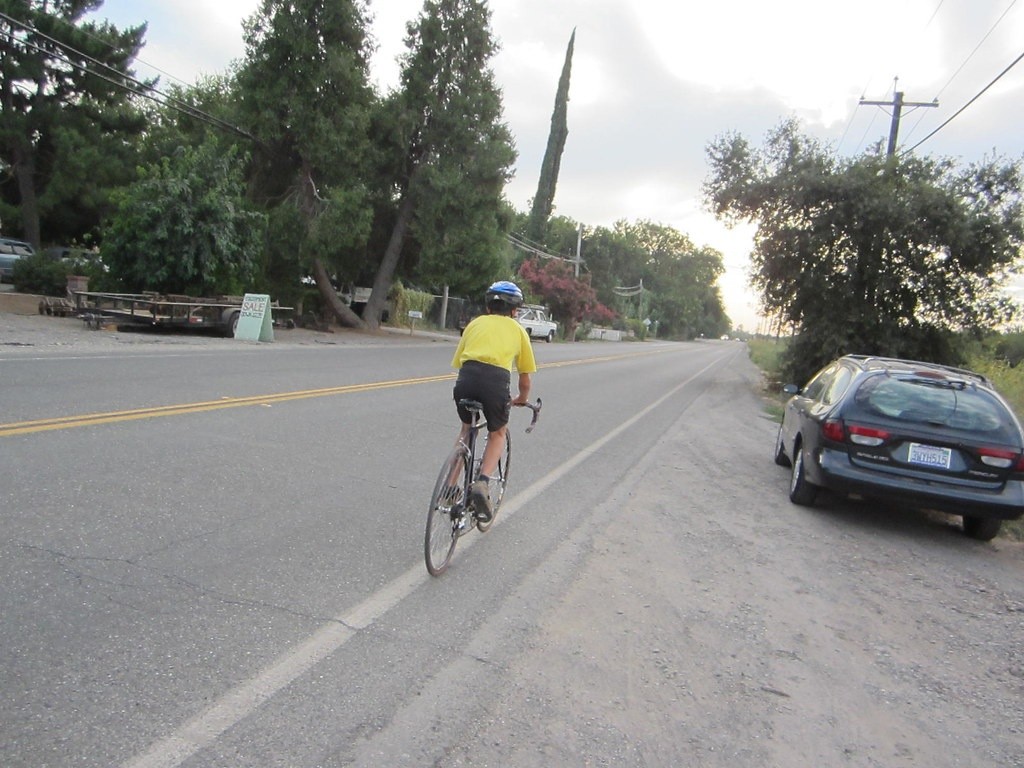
left=774, top=353, right=1024, bottom=541
left=0, top=239, right=37, bottom=284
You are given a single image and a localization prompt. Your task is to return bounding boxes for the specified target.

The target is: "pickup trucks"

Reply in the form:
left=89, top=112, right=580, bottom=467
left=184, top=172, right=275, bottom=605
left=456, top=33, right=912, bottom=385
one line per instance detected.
left=514, top=308, right=557, bottom=342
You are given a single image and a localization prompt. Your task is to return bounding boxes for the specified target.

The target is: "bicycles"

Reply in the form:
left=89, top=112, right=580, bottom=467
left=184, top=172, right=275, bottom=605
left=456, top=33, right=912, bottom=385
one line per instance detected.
left=423, top=399, right=542, bottom=576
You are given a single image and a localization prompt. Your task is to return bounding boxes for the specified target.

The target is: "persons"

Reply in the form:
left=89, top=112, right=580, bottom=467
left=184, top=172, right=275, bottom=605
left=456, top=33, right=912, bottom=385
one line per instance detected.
left=442, top=281, right=536, bottom=524
left=91, top=240, right=99, bottom=251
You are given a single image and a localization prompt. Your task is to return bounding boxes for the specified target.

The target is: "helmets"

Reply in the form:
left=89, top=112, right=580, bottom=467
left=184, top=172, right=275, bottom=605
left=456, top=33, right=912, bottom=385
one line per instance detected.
left=484, top=280, right=523, bottom=307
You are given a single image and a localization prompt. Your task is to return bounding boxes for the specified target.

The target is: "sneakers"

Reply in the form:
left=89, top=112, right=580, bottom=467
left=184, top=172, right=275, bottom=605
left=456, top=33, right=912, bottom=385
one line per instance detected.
left=437, top=486, right=463, bottom=510
left=472, top=481, right=493, bottom=523
